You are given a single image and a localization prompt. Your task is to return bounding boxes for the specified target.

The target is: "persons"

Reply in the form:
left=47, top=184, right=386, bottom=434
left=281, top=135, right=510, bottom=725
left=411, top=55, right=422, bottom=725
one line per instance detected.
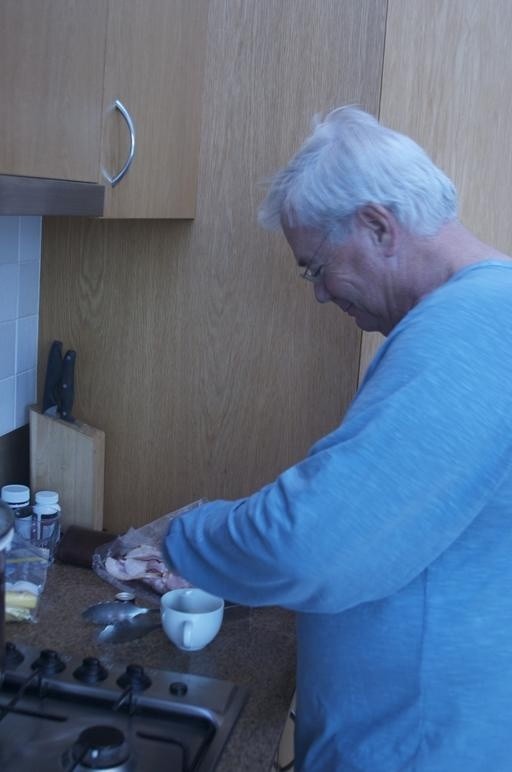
left=168, top=104, right=512, bottom=770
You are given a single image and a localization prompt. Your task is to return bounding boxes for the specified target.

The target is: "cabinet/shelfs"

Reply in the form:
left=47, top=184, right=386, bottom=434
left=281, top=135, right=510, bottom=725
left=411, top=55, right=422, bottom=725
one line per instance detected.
left=0, top=1, right=109, bottom=183
left=99, top=1, right=206, bottom=219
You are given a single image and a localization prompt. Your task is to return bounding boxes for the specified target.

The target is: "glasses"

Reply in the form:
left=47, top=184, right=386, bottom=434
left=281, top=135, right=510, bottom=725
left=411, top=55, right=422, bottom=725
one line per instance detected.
left=302, top=228, right=333, bottom=284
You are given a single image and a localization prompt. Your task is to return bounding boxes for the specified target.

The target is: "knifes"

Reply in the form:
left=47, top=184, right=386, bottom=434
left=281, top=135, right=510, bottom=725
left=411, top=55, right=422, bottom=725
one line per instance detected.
left=42, top=340, right=79, bottom=421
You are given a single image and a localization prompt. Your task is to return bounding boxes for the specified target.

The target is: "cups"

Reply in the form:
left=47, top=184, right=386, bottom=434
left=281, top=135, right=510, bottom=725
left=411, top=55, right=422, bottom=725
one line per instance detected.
left=160, top=588, right=225, bottom=651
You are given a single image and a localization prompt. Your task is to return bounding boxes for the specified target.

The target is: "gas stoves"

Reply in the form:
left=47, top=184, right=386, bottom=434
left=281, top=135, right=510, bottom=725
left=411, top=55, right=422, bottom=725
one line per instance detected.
left=0, top=642, right=249, bottom=772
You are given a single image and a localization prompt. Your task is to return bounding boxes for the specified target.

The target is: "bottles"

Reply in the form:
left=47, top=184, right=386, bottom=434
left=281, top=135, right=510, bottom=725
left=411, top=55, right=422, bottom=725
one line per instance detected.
left=1, top=484, right=36, bottom=544
left=35, top=490, right=62, bottom=547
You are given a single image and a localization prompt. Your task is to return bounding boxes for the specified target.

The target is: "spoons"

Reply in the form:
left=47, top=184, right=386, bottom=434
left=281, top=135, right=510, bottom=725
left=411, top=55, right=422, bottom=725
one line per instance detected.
left=97, top=603, right=235, bottom=643
left=83, top=598, right=160, bottom=625
